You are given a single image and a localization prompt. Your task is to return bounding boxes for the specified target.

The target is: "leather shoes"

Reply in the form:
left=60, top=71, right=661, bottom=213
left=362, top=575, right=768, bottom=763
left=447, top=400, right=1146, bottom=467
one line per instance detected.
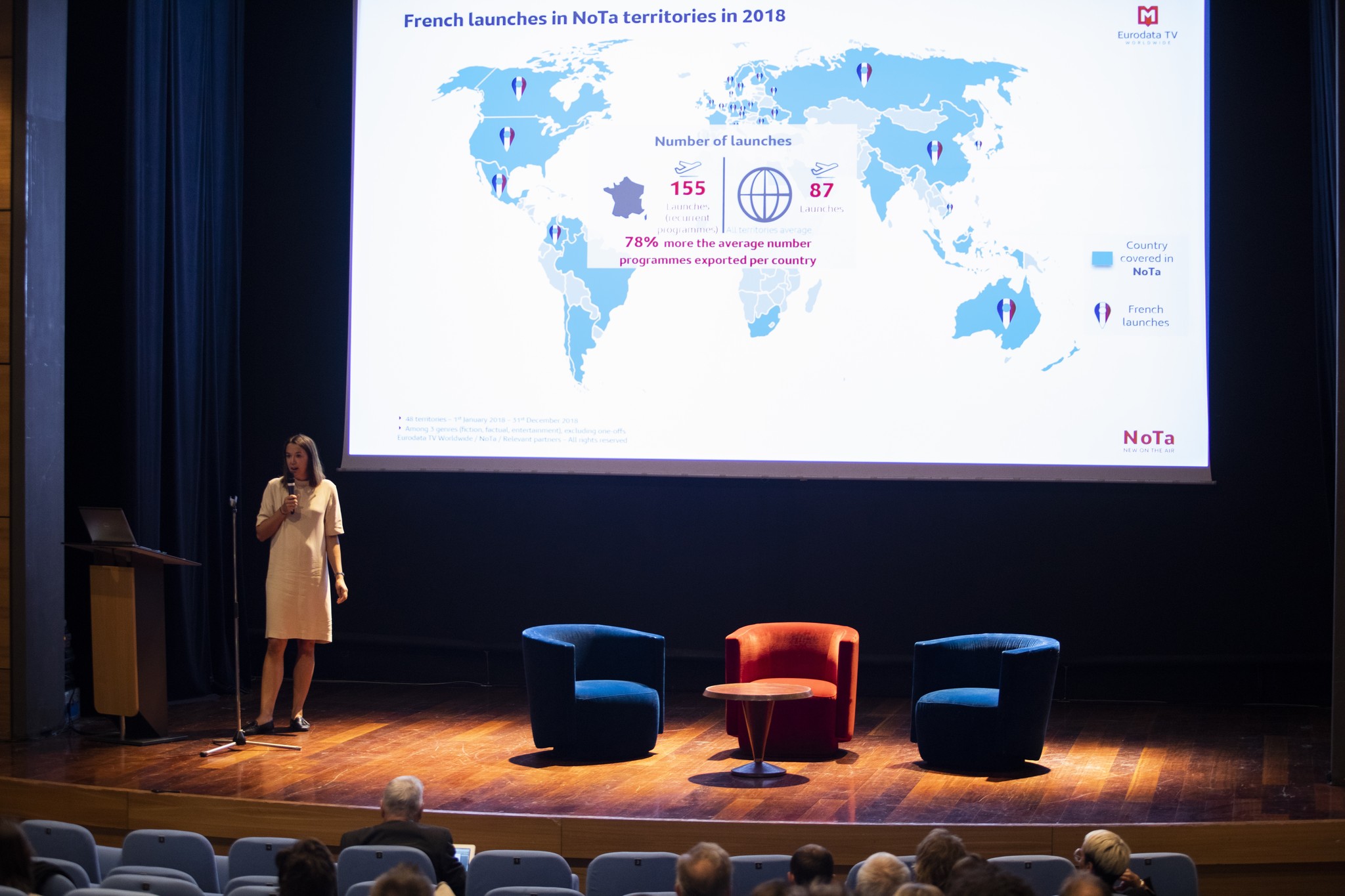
left=290, top=717, right=310, bottom=732
left=241, top=716, right=275, bottom=736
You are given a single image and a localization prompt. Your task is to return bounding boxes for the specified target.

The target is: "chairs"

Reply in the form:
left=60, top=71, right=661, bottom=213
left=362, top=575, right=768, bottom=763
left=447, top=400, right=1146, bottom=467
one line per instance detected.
left=1, top=819, right=1200, bottom=896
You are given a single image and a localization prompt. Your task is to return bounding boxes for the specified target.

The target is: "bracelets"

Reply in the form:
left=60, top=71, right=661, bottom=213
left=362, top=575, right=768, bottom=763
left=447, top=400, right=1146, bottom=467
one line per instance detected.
left=279, top=507, right=288, bottom=514
left=334, top=572, right=344, bottom=578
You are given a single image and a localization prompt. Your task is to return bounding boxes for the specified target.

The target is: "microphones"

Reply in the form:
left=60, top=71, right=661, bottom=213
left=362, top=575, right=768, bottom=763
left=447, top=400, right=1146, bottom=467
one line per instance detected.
left=286, top=471, right=296, bottom=515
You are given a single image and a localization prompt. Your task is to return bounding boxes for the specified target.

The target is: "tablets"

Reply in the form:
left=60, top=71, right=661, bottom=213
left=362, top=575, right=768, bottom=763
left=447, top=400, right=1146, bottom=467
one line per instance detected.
left=451, top=843, right=476, bottom=872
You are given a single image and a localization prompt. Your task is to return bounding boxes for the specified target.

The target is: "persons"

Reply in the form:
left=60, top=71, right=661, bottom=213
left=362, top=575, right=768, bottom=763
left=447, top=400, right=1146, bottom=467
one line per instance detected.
left=275, top=835, right=338, bottom=896
left=369, top=861, right=437, bottom=895
left=240, top=433, right=349, bottom=736
left=340, top=775, right=467, bottom=896
left=674, top=828, right=1154, bottom=896
left=0, top=821, right=44, bottom=896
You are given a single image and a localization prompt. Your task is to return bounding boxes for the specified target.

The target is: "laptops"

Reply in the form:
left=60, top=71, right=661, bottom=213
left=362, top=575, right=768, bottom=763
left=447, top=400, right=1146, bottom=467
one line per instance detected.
left=78, top=506, right=161, bottom=553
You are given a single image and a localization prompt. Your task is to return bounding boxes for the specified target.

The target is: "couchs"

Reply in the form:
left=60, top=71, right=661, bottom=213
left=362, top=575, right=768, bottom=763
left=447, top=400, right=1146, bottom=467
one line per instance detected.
left=724, top=621, right=858, bottom=757
left=909, top=634, right=1061, bottom=773
left=522, top=622, right=666, bottom=759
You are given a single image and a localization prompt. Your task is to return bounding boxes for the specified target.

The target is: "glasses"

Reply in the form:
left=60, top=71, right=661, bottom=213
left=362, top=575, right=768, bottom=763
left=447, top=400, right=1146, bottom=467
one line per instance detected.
left=1073, top=848, right=1082, bottom=863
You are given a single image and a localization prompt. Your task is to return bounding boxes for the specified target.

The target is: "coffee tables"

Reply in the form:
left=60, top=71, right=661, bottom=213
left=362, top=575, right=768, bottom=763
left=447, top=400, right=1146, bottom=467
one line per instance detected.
left=702, top=684, right=813, bottom=779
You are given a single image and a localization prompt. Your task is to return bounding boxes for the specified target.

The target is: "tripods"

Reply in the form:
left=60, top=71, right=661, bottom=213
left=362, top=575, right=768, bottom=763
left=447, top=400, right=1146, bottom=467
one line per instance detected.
left=200, top=495, right=301, bottom=759
